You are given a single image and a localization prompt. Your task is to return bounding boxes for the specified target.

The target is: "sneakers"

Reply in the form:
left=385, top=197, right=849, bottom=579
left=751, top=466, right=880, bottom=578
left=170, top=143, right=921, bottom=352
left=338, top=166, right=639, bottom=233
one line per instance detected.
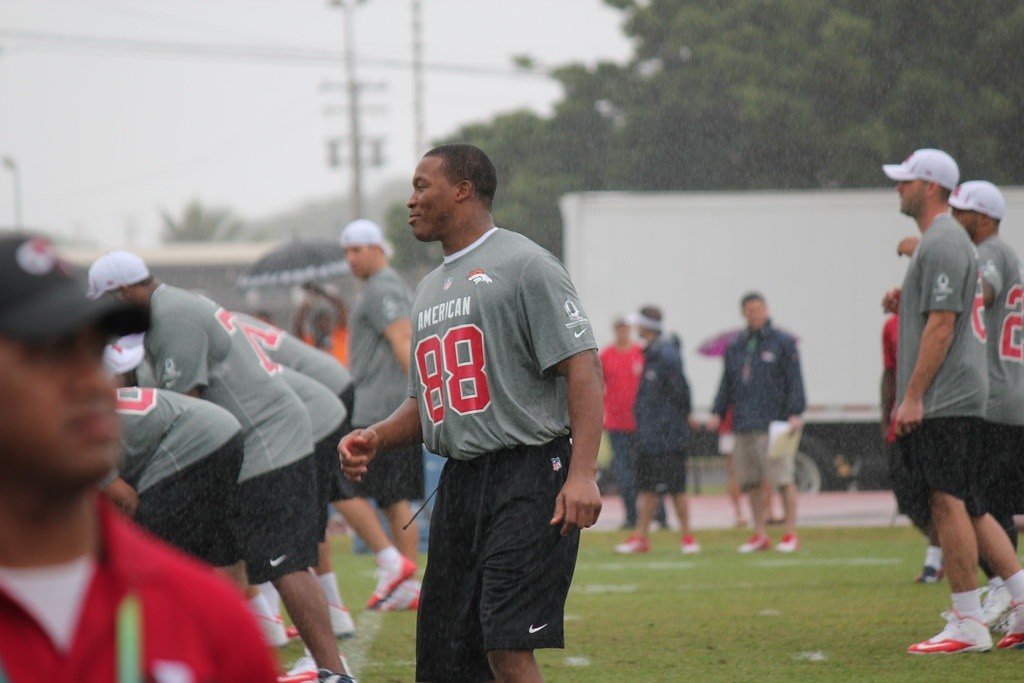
left=977, top=583, right=1011, bottom=626
left=681, top=534, right=700, bottom=555
left=737, top=532, right=772, bottom=552
left=993, top=612, right=1015, bottom=634
left=377, top=580, right=421, bottom=611
left=776, top=531, right=797, bottom=552
left=996, top=594, right=1024, bottom=650
left=258, top=612, right=289, bottom=646
left=285, top=602, right=353, bottom=639
left=364, top=552, right=416, bottom=610
left=907, top=610, right=993, bottom=655
left=915, top=565, right=945, bottom=585
left=309, top=667, right=355, bottom=683
left=276, top=648, right=349, bottom=682
left=613, top=536, right=648, bottom=555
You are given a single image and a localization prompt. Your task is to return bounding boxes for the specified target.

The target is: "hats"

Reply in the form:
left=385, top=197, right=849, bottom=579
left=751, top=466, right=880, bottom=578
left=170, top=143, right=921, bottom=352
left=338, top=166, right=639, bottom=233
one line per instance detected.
left=883, top=148, right=960, bottom=191
left=948, top=180, right=1003, bottom=220
left=116, top=332, right=144, bottom=349
left=101, top=344, right=144, bottom=373
left=85, top=251, right=151, bottom=301
left=342, top=219, right=391, bottom=260
left=626, top=309, right=664, bottom=331
left=0, top=230, right=153, bottom=347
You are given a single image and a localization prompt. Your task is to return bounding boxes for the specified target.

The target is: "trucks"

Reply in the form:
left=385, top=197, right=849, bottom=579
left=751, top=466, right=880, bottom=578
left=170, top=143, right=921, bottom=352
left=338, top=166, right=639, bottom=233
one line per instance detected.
left=557, top=184, right=1023, bottom=500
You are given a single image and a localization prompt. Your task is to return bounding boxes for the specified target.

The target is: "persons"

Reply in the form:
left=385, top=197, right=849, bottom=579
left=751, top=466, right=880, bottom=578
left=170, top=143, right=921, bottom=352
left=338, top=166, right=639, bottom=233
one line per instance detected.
left=0, top=230, right=285, bottom=683
left=878, top=148, right=1023, bottom=653
left=703, top=294, right=809, bottom=552
left=337, top=143, right=605, bottom=683
left=95, top=386, right=246, bottom=564
left=83, top=252, right=362, bottom=683
left=327, top=220, right=425, bottom=612
left=594, top=305, right=703, bottom=557
